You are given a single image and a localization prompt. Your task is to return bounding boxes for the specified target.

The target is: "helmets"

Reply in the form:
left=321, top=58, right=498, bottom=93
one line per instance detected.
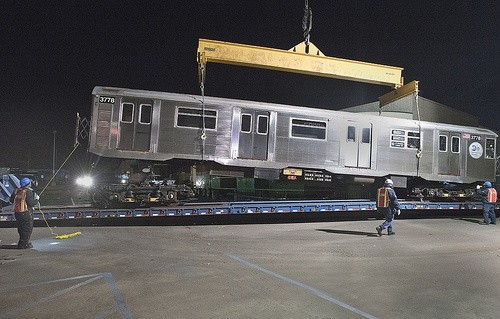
left=385, top=179, right=393, bottom=184
left=20, top=178, right=32, bottom=186
left=483, top=181, right=492, bottom=187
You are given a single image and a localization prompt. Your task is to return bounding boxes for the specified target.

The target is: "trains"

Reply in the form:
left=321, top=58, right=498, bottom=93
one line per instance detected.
left=90, top=85, right=499, bottom=201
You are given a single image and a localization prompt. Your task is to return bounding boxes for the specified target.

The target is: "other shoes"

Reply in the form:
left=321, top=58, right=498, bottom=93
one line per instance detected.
left=20, top=245, right=33, bottom=248
left=490, top=221, right=494, bottom=224
left=483, top=222, right=489, bottom=224
left=376, top=226, right=382, bottom=236
left=388, top=232, right=395, bottom=235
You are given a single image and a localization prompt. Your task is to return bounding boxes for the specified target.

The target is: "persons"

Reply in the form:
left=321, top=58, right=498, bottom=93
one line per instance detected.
left=476, top=181, right=497, bottom=225
left=375, top=179, right=400, bottom=236
left=10, top=177, right=40, bottom=248
